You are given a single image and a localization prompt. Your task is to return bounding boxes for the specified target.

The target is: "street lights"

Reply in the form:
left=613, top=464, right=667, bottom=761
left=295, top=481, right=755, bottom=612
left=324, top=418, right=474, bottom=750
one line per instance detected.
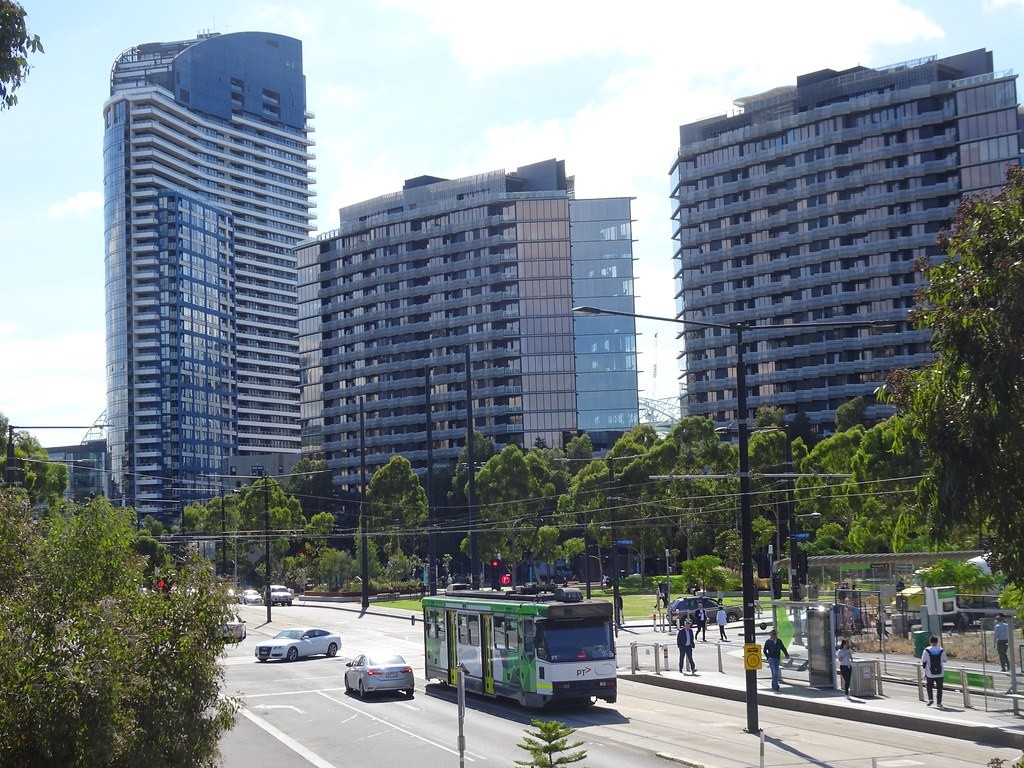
left=162, top=482, right=278, bottom=576
left=195, top=470, right=330, bottom=622
left=553, top=454, right=643, bottom=630
left=714, top=424, right=821, bottom=600
left=570, top=304, right=905, bottom=733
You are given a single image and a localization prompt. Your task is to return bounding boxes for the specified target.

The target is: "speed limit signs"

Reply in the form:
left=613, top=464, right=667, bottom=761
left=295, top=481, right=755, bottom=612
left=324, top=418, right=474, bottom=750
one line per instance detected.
left=747, top=654, right=759, bottom=667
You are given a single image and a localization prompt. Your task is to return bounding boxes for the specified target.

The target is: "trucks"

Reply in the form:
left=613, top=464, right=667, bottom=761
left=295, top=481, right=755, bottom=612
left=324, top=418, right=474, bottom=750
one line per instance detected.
left=889, top=552, right=1005, bottom=631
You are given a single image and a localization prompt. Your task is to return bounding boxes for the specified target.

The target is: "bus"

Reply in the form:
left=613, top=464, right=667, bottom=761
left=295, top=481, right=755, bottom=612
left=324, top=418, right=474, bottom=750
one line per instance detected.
left=423, top=582, right=619, bottom=711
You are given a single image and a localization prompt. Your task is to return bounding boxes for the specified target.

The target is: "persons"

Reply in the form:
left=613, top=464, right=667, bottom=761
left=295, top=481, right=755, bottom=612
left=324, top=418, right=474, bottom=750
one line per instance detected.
left=695, top=604, right=709, bottom=641
left=851, top=585, right=858, bottom=599
left=837, top=639, right=852, bottom=699
left=654, top=582, right=668, bottom=608
left=921, top=636, right=946, bottom=707
left=875, top=606, right=890, bottom=637
left=677, top=622, right=697, bottom=673
left=717, top=606, right=727, bottom=640
left=763, top=630, right=789, bottom=691
left=896, top=578, right=904, bottom=592
left=993, top=616, right=1011, bottom=671
left=948, top=613, right=968, bottom=635
left=717, top=590, right=723, bottom=604
left=691, top=584, right=700, bottom=596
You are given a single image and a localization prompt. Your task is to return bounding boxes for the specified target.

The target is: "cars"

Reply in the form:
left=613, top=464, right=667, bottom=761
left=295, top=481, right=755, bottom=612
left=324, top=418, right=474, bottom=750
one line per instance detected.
left=217, top=615, right=246, bottom=642
left=254, top=628, right=342, bottom=663
left=344, top=653, right=414, bottom=699
left=787, top=602, right=871, bottom=639
left=239, top=590, right=262, bottom=605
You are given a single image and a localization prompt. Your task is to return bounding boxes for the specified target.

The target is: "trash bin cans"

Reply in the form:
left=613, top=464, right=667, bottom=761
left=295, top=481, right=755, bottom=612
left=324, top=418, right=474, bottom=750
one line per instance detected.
left=914, top=631, right=931, bottom=658
left=850, top=660, right=876, bottom=697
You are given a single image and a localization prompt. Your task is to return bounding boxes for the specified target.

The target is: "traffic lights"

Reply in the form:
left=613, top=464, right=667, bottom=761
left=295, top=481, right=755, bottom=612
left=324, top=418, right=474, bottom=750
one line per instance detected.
left=491, top=559, right=501, bottom=591
left=156, top=576, right=171, bottom=593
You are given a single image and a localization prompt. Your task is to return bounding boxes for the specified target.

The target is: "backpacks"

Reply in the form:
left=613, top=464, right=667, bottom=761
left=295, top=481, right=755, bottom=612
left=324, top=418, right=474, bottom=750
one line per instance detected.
left=925, top=649, right=943, bottom=674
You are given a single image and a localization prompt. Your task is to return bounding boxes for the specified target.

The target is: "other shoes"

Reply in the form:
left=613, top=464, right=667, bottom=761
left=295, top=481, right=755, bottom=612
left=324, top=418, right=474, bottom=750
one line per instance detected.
left=724, top=634, right=727, bottom=639
left=703, top=639, right=706, bottom=642
left=845, top=694, right=851, bottom=700
left=719, top=638, right=723, bottom=640
left=927, top=700, right=933, bottom=706
left=692, top=669, right=697, bottom=673
left=938, top=704, right=943, bottom=707
left=695, top=635, right=697, bottom=640
left=1001, top=668, right=1006, bottom=672
left=772, top=684, right=779, bottom=691
left=679, top=667, right=682, bottom=673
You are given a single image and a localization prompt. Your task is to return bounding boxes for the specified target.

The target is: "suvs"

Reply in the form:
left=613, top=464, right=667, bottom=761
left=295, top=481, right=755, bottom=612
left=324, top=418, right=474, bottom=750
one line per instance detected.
left=264, top=585, right=292, bottom=606
left=668, top=595, right=743, bottom=629
